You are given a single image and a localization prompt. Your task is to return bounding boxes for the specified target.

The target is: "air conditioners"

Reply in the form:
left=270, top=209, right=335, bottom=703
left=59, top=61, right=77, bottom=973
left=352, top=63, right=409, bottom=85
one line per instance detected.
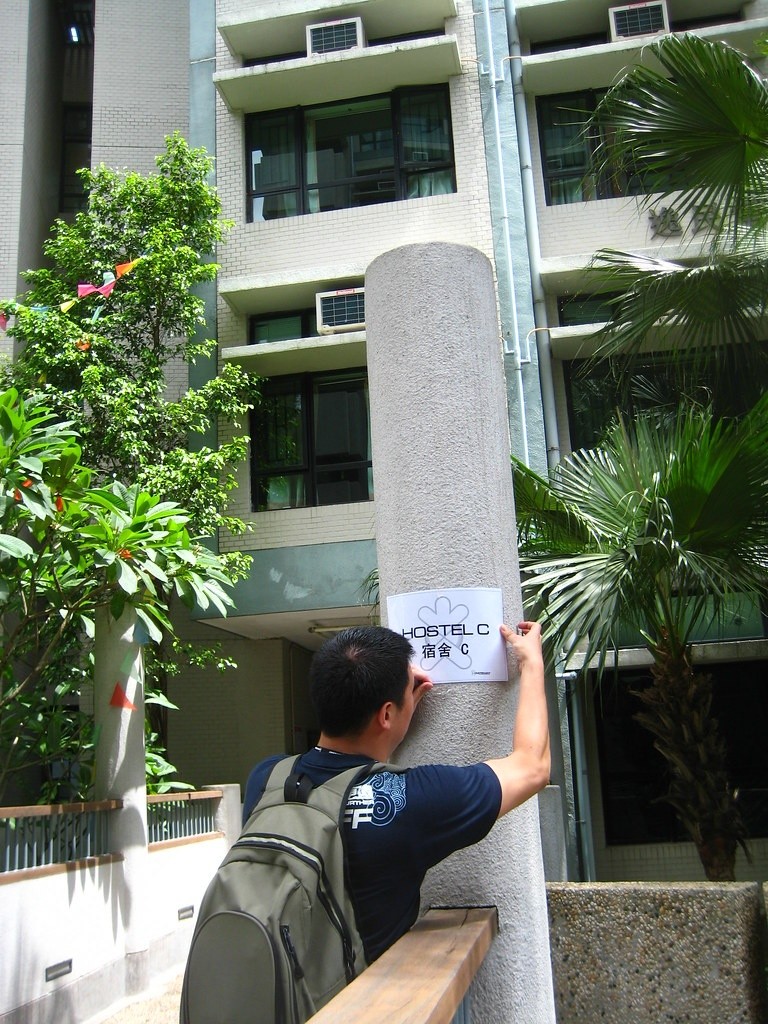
left=306, top=16, right=368, bottom=57
left=607, top=0, right=671, bottom=42
left=315, top=287, right=365, bottom=336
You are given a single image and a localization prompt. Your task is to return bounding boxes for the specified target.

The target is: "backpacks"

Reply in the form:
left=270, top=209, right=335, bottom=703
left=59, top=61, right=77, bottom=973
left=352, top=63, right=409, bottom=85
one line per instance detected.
left=179, top=751, right=413, bottom=1024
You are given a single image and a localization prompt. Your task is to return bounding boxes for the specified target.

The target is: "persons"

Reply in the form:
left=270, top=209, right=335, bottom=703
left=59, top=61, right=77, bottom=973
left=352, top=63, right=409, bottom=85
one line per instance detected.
left=245, top=621, right=555, bottom=967
